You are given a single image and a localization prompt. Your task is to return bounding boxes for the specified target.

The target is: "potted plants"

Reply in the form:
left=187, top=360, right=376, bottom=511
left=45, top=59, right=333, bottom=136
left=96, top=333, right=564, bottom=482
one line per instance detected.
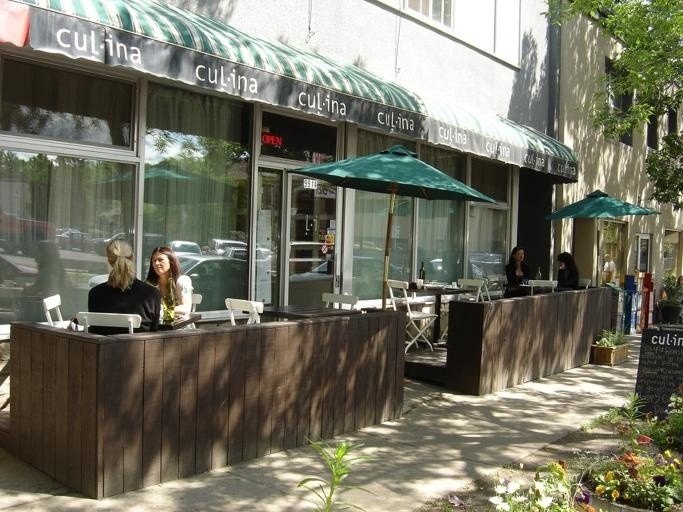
left=589, top=326, right=629, bottom=365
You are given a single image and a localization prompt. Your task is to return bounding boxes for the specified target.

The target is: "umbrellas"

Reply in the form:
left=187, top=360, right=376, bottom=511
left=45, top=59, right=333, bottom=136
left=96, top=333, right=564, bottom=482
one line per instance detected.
left=286, top=145, right=498, bottom=309
left=545, top=190, right=661, bottom=286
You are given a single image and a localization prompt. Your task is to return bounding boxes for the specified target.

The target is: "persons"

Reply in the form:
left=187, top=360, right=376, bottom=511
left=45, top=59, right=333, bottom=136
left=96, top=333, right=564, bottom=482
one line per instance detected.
left=504, top=247, right=530, bottom=298
left=25, top=240, right=65, bottom=296
left=604, top=254, right=616, bottom=283
left=557, top=252, right=579, bottom=287
left=88, top=239, right=196, bottom=335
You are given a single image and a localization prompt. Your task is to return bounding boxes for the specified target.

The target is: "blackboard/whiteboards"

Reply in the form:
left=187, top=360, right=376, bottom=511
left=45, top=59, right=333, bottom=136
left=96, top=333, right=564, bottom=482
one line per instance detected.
left=633, top=328, right=683, bottom=422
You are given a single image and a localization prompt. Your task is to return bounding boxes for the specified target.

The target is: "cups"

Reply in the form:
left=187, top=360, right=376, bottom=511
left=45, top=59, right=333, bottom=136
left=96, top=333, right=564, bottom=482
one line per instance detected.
left=522, top=278, right=527, bottom=285
left=452, top=282, right=456, bottom=288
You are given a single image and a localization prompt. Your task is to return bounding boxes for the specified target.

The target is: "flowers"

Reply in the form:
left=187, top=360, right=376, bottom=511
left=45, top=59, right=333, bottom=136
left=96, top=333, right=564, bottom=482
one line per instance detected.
left=584, top=450, right=682, bottom=509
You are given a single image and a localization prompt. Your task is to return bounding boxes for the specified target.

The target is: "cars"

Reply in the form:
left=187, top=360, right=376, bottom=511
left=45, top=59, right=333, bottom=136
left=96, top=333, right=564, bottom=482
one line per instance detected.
left=54, top=227, right=274, bottom=310
left=0, top=210, right=55, bottom=257
left=288, top=253, right=506, bottom=304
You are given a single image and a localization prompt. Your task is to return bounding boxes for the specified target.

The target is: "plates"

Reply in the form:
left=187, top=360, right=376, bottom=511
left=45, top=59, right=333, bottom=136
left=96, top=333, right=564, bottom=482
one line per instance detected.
left=423, top=285, right=445, bottom=289
left=446, top=288, right=460, bottom=290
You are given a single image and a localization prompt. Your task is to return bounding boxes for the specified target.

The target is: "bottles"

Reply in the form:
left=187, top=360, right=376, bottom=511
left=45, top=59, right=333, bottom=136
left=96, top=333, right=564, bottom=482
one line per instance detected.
left=533, top=266, right=542, bottom=279
left=419, top=260, right=426, bottom=283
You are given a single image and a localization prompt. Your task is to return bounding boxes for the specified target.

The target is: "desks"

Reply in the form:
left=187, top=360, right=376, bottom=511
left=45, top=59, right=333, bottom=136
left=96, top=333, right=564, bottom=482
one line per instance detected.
left=518, top=283, right=574, bottom=295
left=240, top=303, right=362, bottom=321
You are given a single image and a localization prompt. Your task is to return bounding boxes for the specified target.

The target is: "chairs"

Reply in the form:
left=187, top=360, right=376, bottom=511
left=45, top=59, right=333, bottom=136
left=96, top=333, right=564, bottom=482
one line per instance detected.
left=40, top=290, right=141, bottom=337
left=527, top=279, right=558, bottom=295
left=319, top=291, right=358, bottom=310
left=223, top=297, right=263, bottom=324
left=386, top=278, right=491, bottom=354
